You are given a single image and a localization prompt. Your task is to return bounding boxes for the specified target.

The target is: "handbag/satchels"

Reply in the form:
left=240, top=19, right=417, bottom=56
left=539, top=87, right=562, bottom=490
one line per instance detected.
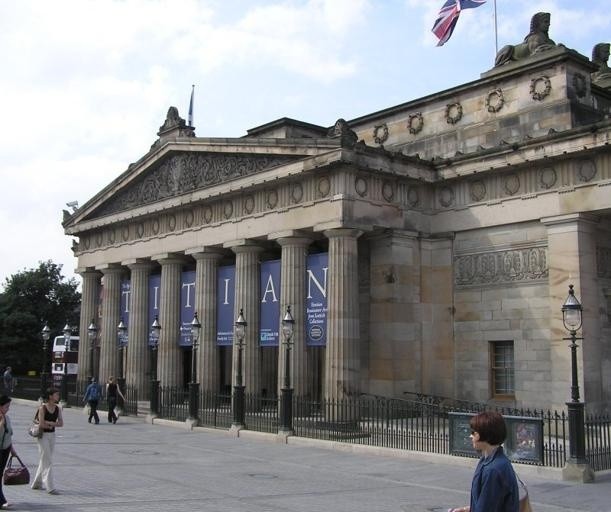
left=4, top=467, right=30, bottom=485
left=82, top=404, right=92, bottom=415
left=27, top=419, right=40, bottom=437
left=519, top=494, right=533, bottom=512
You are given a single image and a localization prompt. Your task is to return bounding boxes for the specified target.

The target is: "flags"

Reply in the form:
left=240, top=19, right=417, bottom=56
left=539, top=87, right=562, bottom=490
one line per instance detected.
left=430, top=1, right=488, bottom=48
left=188, top=84, right=196, bottom=127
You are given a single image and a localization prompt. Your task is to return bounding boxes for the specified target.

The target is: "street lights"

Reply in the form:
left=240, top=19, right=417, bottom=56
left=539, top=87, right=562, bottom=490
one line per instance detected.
left=560, top=284, right=591, bottom=466
left=150, top=315, right=162, bottom=414
left=40, top=320, right=53, bottom=398
left=188, top=311, right=202, bottom=420
left=116, top=317, right=128, bottom=409
left=86, top=318, right=99, bottom=406
left=61, top=319, right=73, bottom=402
left=279, top=306, right=296, bottom=431
left=232, top=309, right=248, bottom=425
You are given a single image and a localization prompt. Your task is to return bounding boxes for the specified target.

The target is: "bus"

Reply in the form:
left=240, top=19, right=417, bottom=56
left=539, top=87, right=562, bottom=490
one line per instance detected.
left=50, top=335, right=79, bottom=392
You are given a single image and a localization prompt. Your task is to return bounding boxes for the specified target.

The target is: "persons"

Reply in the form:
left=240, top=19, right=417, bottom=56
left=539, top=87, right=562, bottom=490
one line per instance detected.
left=2, top=365, right=15, bottom=397
left=591, top=43, right=611, bottom=80
left=445, top=408, right=521, bottom=512
left=0, top=395, right=16, bottom=511
left=81, top=376, right=104, bottom=425
left=494, top=10, right=558, bottom=68
left=105, top=376, right=127, bottom=424
left=30, top=387, right=66, bottom=495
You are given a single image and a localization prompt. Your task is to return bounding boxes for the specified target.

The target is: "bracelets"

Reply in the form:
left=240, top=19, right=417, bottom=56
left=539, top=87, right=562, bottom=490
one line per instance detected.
left=464, top=506, right=467, bottom=512
